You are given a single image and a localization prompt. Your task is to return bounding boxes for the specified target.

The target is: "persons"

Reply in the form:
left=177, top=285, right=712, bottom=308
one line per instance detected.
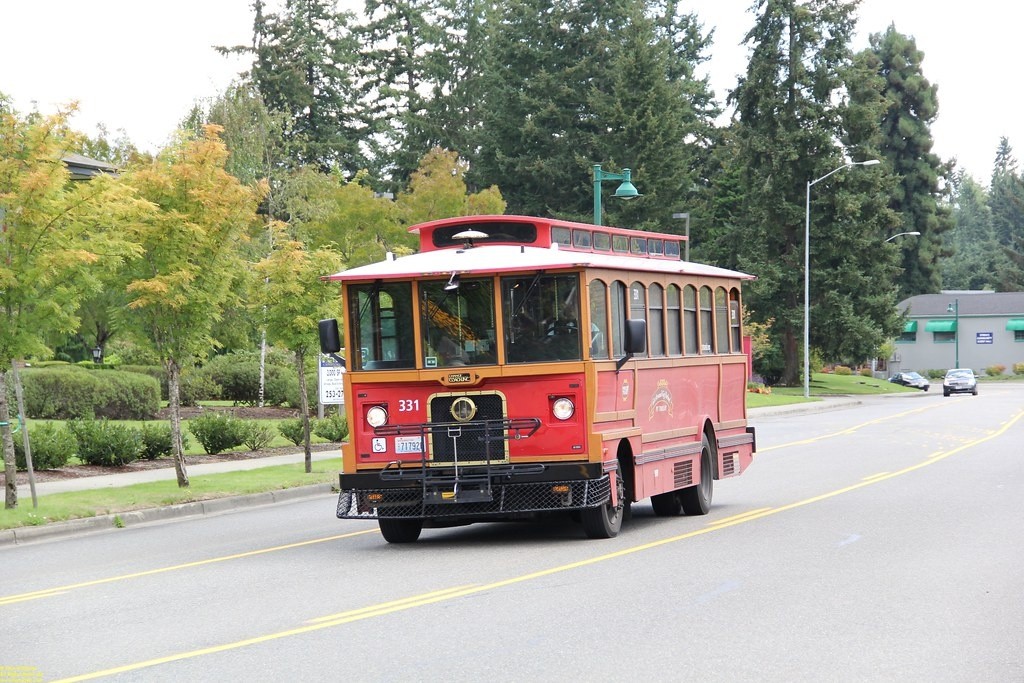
left=544, top=293, right=604, bottom=354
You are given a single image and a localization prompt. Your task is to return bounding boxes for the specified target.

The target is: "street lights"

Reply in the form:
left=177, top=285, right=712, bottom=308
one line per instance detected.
left=871, top=231, right=923, bottom=379
left=804, top=159, right=881, bottom=396
left=591, top=164, right=643, bottom=226
left=947, top=298, right=959, bottom=368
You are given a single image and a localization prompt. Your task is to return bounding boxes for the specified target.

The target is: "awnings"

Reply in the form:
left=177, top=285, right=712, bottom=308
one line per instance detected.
left=900, top=321, right=917, bottom=332
left=1006, top=319, right=1024, bottom=331
left=925, top=320, right=957, bottom=332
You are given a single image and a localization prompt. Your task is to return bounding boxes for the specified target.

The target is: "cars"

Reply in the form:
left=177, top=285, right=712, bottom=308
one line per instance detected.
left=887, top=372, right=930, bottom=392
left=940, top=368, right=979, bottom=396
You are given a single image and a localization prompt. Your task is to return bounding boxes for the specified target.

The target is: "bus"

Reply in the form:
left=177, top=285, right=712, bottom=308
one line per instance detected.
left=318, top=215, right=757, bottom=543
